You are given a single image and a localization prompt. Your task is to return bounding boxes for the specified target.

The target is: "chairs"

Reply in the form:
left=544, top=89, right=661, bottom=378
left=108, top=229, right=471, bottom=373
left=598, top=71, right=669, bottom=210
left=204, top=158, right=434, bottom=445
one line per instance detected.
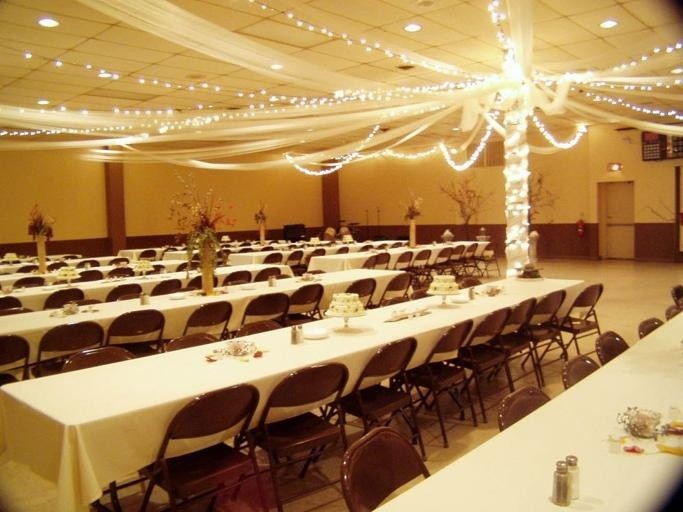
left=495, top=290, right=568, bottom=387
left=264, top=253, right=282, bottom=264
left=183, top=301, right=232, bottom=341
left=32, top=321, right=104, bottom=378
left=665, top=305, right=683, bottom=320
left=106, top=309, right=165, bottom=357
left=393, top=252, right=413, bottom=272
left=475, top=243, right=501, bottom=279
left=261, top=240, right=336, bottom=251
left=346, top=278, right=376, bottom=310
left=275, top=284, right=324, bottom=327
left=390, top=319, right=478, bottom=449
left=0, top=335, right=29, bottom=386
left=295, top=249, right=325, bottom=276
left=105, top=258, right=251, bottom=303
left=362, top=256, right=377, bottom=269
left=331, top=340, right=427, bottom=457
left=458, top=243, right=483, bottom=279
left=409, top=250, right=431, bottom=276
left=449, top=245, right=465, bottom=275
left=411, top=292, right=430, bottom=300
left=378, top=274, right=413, bottom=306
left=458, top=277, right=482, bottom=290
left=285, top=251, right=303, bottom=277
left=596, top=331, right=629, bottom=365
left=233, top=363, right=348, bottom=512
left=539, top=283, right=602, bottom=362
left=562, top=356, right=599, bottom=388
left=383, top=296, right=410, bottom=306
left=165, top=332, right=219, bottom=354
left=138, top=383, right=272, bottom=512
left=432, top=247, right=454, bottom=274
left=341, top=426, right=431, bottom=512
left=638, top=318, right=664, bottom=338
left=444, top=307, right=513, bottom=423
left=137, top=241, right=261, bottom=260
left=498, top=388, right=549, bottom=433
left=60, top=347, right=137, bottom=374
left=237, top=320, right=281, bottom=339
left=0, top=256, right=103, bottom=317
left=241, top=294, right=289, bottom=326
left=489, top=298, right=542, bottom=393
left=671, top=285, right=683, bottom=310
left=254, top=267, right=325, bottom=282
left=375, top=252, right=391, bottom=269
left=336, top=240, right=410, bottom=254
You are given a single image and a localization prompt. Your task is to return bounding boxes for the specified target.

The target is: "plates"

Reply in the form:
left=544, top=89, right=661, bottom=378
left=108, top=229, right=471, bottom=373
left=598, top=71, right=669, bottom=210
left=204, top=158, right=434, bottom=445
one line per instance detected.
left=302, top=329, right=329, bottom=340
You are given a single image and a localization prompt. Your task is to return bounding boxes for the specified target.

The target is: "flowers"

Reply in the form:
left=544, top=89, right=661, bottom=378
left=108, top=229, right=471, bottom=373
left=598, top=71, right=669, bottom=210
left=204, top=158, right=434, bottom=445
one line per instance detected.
left=254, top=203, right=268, bottom=223
left=27, top=204, right=54, bottom=246
left=169, top=170, right=235, bottom=280
left=403, top=200, right=421, bottom=220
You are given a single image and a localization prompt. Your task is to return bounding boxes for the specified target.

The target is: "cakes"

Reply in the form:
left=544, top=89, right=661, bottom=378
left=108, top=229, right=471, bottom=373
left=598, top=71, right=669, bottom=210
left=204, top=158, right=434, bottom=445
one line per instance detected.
left=342, top=235, right=353, bottom=241
left=221, top=235, right=230, bottom=241
left=310, top=238, right=319, bottom=243
left=328, top=293, right=364, bottom=315
left=57, top=267, right=78, bottom=277
left=442, top=228, right=454, bottom=237
left=429, top=275, right=458, bottom=293
left=3, top=253, right=17, bottom=260
left=135, top=260, right=153, bottom=271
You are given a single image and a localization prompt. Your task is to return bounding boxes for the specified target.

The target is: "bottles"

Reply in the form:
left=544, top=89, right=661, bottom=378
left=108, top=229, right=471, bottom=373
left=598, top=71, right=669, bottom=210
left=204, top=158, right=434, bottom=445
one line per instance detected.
left=290, top=323, right=304, bottom=345
left=140, top=291, right=149, bottom=306
left=268, top=275, right=276, bottom=286
left=566, top=456, right=579, bottom=500
left=552, top=461, right=572, bottom=506
left=469, top=287, right=475, bottom=299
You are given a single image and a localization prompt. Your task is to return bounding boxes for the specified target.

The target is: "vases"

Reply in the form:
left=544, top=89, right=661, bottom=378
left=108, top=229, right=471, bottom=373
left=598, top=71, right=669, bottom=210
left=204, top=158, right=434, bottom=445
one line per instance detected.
left=410, top=220, right=416, bottom=248
left=34, top=233, right=48, bottom=274
left=196, top=245, right=216, bottom=295
left=260, top=223, right=265, bottom=246
